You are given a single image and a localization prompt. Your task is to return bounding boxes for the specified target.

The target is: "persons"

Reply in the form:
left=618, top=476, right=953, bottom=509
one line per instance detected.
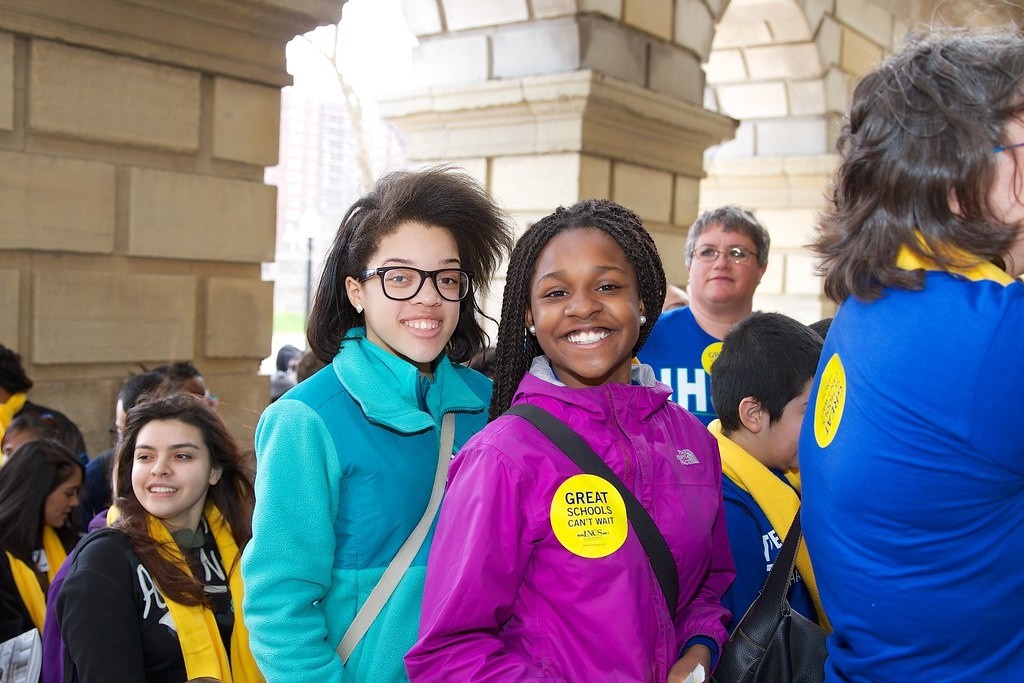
left=1, top=343, right=302, bottom=683
left=705, top=312, right=836, bottom=683
left=404, top=198, right=735, bottom=682
left=240, top=165, right=515, bottom=683
left=634, top=204, right=769, bottom=427
left=798, top=26, right=1024, bottom=682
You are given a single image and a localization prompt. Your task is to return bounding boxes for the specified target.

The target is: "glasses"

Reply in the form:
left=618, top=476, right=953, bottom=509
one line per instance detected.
left=693, top=243, right=759, bottom=265
left=356, top=266, right=476, bottom=301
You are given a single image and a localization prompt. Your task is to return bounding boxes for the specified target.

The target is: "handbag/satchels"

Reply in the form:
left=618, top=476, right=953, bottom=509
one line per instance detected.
left=710, top=592, right=828, bottom=683
